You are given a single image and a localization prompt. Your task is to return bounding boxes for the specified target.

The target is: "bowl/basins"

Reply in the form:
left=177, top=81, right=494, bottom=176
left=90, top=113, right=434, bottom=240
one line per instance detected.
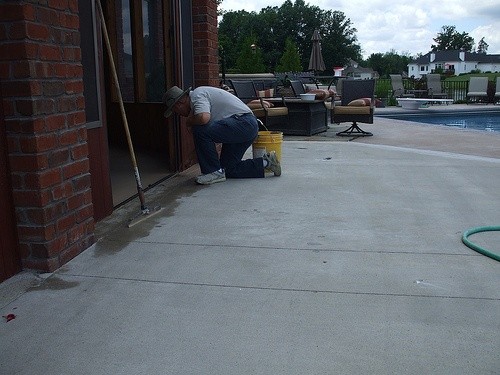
left=300, top=93, right=316, bottom=101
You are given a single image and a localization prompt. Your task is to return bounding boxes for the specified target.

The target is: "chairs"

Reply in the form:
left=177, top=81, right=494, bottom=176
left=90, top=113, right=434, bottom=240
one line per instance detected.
left=427, top=74, right=448, bottom=106
left=494, top=77, right=500, bottom=104
left=388, top=74, right=416, bottom=98
left=467, top=77, right=489, bottom=105
left=227, top=79, right=376, bottom=137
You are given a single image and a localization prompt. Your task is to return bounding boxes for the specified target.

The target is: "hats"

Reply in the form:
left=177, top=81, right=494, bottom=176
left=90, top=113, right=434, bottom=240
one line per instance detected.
left=162, top=86, right=192, bottom=118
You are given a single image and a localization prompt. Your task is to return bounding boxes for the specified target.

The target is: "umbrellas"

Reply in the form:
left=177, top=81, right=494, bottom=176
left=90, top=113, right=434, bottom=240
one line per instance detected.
left=308, top=31, right=326, bottom=75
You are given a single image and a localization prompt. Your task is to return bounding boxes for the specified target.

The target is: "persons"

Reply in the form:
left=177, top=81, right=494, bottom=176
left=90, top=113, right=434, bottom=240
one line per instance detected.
left=162, top=86, right=281, bottom=184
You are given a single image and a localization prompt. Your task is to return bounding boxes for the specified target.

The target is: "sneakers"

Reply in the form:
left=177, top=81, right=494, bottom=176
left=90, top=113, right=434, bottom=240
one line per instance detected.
left=262, top=151, right=282, bottom=176
left=192, top=169, right=226, bottom=185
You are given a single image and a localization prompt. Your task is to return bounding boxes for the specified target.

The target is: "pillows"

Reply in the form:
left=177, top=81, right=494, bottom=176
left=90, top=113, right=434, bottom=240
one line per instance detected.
left=306, top=88, right=336, bottom=100
left=246, top=100, right=274, bottom=109
left=347, top=98, right=375, bottom=106
left=256, top=88, right=274, bottom=98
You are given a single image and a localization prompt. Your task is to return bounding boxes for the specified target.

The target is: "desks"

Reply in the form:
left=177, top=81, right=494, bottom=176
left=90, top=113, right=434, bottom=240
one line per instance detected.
left=260, top=97, right=328, bottom=137
left=299, top=76, right=350, bottom=90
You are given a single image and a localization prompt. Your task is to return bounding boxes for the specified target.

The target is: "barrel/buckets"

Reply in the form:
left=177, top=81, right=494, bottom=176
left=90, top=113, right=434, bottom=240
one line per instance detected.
left=252, top=118, right=283, bottom=173
